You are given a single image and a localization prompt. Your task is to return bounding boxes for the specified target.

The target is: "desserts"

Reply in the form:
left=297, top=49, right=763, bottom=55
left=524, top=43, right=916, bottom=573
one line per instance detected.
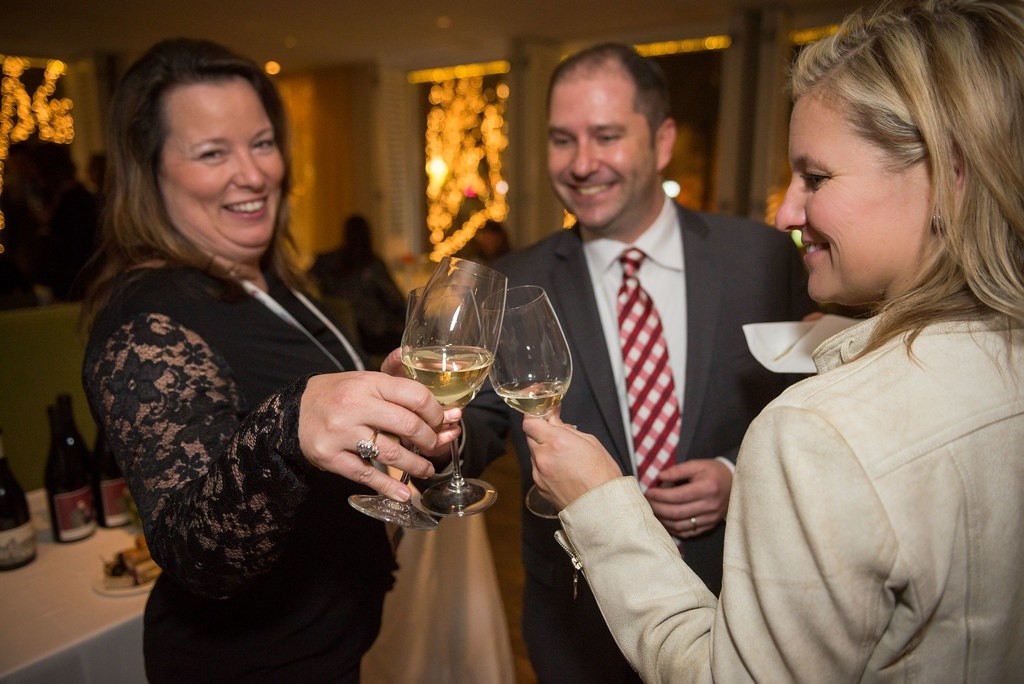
left=104, top=546, right=162, bottom=587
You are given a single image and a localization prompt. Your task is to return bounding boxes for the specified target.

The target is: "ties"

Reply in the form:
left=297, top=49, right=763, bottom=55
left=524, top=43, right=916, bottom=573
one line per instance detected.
left=616, top=247, right=682, bottom=498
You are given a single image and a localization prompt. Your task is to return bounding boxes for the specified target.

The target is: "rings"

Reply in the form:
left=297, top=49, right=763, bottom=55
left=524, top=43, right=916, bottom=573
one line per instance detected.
left=690, top=517, right=698, bottom=531
left=355, top=429, right=380, bottom=461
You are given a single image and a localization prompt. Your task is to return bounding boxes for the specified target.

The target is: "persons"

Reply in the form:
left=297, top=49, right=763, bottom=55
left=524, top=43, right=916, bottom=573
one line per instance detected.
left=77, top=33, right=446, bottom=684
left=306, top=214, right=516, bottom=358
left=0, top=139, right=111, bottom=309
left=383, top=45, right=817, bottom=684
left=523, top=0, right=1024, bottom=684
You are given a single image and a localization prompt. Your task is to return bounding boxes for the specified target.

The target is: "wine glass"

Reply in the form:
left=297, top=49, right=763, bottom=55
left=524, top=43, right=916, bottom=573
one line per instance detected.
left=481, top=286, right=574, bottom=520
left=349, top=257, right=508, bottom=531
left=404, top=285, right=498, bottom=518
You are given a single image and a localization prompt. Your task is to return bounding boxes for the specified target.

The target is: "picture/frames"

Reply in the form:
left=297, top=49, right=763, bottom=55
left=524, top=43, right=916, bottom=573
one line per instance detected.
left=550, top=9, right=755, bottom=232
left=765, top=15, right=872, bottom=231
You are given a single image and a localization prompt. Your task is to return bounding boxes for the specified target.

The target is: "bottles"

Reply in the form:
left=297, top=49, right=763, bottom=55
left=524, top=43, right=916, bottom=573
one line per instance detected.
left=43, top=392, right=136, bottom=543
left=0, top=428, right=37, bottom=572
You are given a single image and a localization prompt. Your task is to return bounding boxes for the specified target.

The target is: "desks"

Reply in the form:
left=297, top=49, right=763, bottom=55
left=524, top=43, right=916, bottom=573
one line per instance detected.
left=0, top=465, right=515, bottom=684
